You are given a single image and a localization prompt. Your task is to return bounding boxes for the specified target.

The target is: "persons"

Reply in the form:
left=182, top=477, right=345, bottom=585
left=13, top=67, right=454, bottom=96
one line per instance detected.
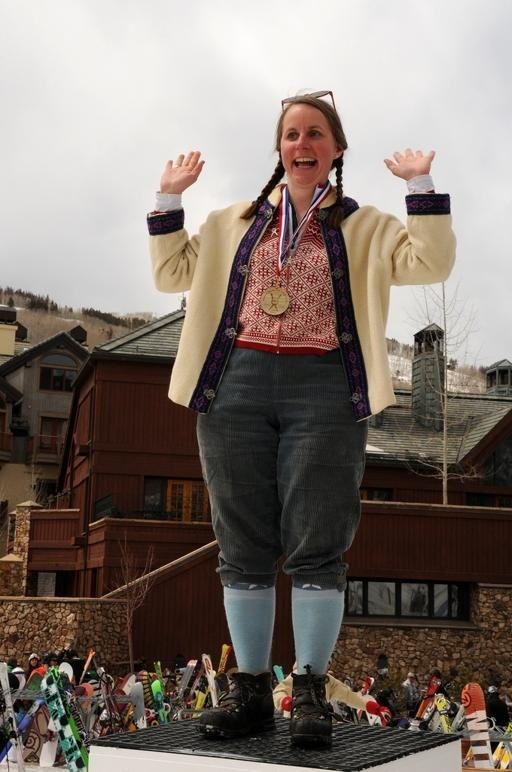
left=144, top=86, right=458, bottom=755
left=1, top=649, right=512, bottom=772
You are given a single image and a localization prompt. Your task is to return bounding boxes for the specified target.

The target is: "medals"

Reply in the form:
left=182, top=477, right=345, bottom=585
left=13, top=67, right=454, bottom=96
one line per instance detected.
left=260, top=280, right=291, bottom=319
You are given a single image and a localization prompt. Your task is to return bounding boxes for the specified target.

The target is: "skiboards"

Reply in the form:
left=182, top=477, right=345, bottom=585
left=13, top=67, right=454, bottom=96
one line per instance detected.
left=342, top=670, right=497, bottom=769
left=0, top=644, right=236, bottom=772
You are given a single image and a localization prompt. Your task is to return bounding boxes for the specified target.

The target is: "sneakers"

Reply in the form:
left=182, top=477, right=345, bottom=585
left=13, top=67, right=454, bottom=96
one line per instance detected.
left=197, top=671, right=276, bottom=739
left=289, top=671, right=349, bottom=747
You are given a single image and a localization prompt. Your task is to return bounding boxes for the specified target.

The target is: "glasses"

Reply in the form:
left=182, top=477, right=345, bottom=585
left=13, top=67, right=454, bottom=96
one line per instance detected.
left=281, top=90, right=335, bottom=110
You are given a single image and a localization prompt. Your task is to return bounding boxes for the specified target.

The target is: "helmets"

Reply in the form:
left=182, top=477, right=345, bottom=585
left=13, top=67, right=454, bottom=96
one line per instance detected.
left=29, top=653, right=40, bottom=662
left=488, top=686, right=498, bottom=695
